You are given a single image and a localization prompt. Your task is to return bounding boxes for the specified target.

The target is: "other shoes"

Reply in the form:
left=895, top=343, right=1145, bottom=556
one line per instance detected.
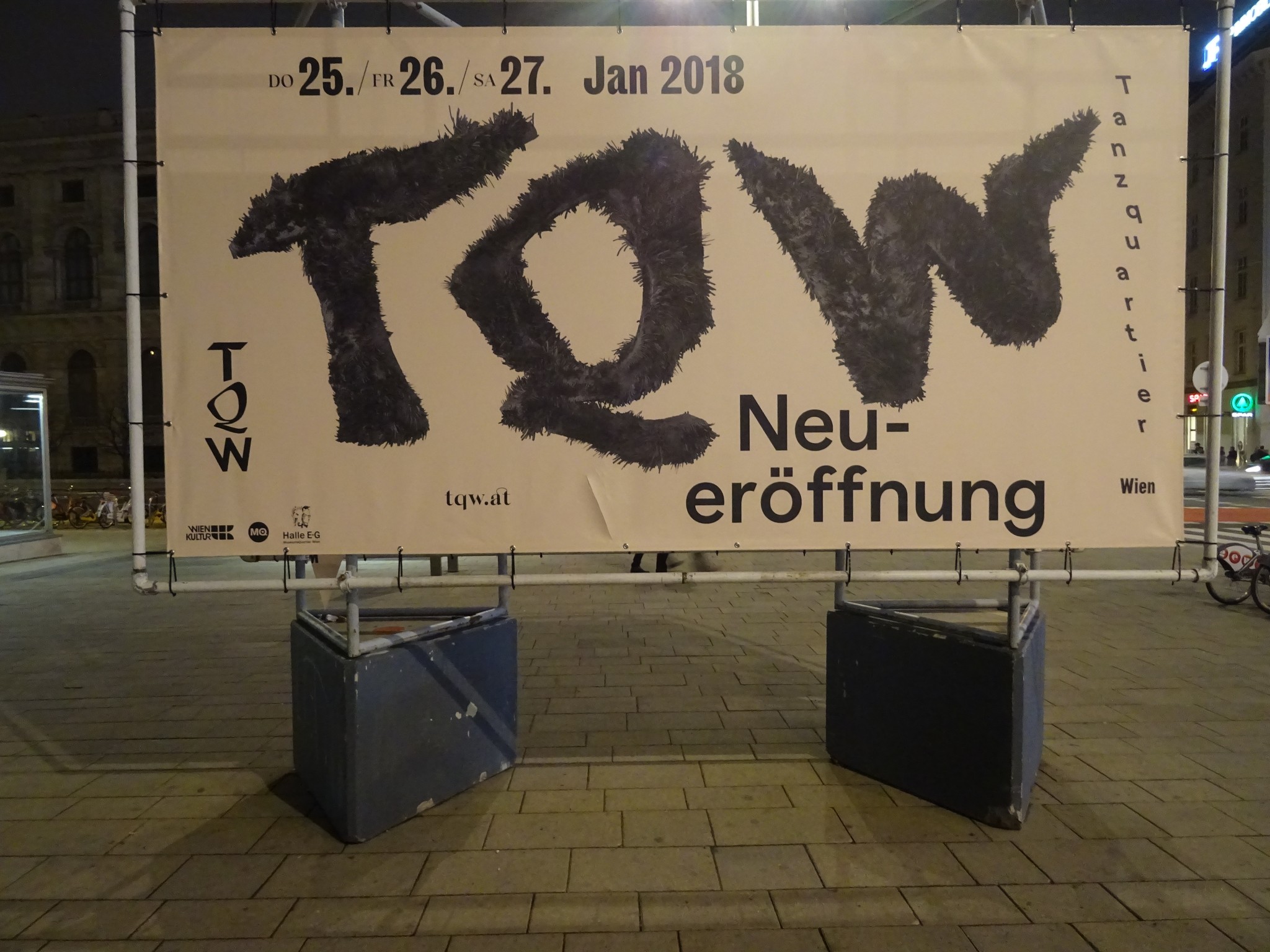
left=630, top=567, right=650, bottom=573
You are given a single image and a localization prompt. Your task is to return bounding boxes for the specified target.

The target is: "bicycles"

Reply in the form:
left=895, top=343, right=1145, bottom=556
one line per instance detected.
left=0, top=483, right=167, bottom=529
left=1205, top=525, right=1270, bottom=612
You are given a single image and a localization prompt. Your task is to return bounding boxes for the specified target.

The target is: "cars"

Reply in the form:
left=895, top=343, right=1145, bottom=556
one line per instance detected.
left=1182, top=453, right=1270, bottom=492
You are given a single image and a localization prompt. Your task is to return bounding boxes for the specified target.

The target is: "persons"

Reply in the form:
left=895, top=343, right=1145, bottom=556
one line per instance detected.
left=1227, top=446, right=1237, bottom=466
left=630, top=553, right=669, bottom=573
left=1194, top=442, right=1204, bottom=454
left=1250, top=445, right=1269, bottom=462
left=1220, top=446, right=1226, bottom=455
